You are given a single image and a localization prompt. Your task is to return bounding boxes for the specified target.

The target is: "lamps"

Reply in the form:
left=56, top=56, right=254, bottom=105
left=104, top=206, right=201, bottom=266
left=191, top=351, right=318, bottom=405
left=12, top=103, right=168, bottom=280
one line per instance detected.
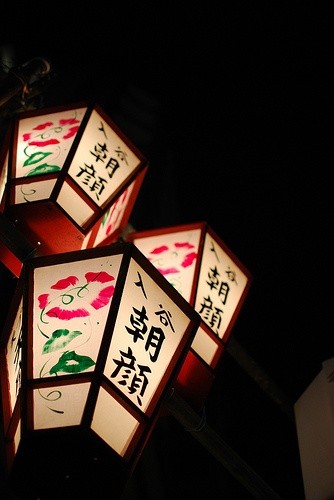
left=0, top=105, right=149, bottom=277
left=1, top=240, right=200, bottom=489
left=126, top=219, right=249, bottom=391
